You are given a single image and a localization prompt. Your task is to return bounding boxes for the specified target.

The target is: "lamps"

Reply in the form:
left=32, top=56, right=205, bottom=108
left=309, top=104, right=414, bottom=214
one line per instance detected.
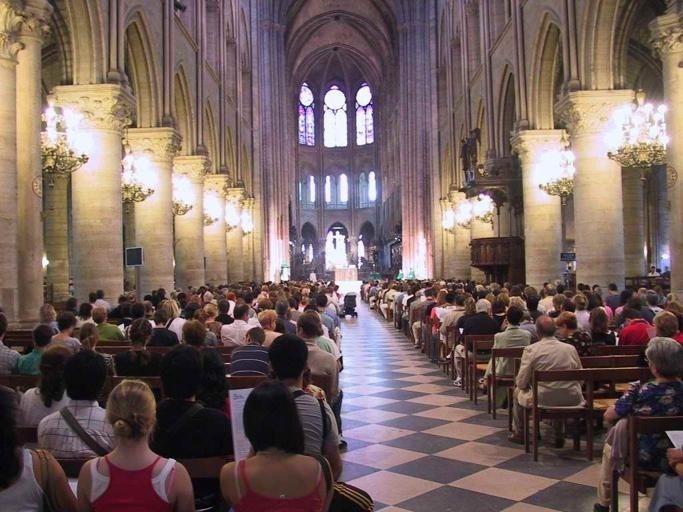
left=439, top=101, right=668, bottom=234
left=41, top=102, right=254, bottom=236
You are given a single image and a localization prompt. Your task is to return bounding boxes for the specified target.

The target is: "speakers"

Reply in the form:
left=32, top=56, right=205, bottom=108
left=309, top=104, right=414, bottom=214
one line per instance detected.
left=125, top=247, right=144, bottom=267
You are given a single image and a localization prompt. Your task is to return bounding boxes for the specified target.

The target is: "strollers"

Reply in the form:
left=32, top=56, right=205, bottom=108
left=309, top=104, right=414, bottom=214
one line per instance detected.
left=337, top=291, right=358, bottom=319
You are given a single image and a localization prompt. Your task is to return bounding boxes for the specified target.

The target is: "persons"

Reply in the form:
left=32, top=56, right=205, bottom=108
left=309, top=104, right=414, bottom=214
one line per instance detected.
left=75, top=380, right=196, bottom=512
left=36, top=349, right=116, bottom=460
left=506, top=315, right=585, bottom=448
left=1, top=279, right=347, bottom=451
left=592, top=337, right=682, bottom=511
left=648, top=266, right=660, bottom=276
left=647, top=447, right=682, bottom=511
left=661, top=266, right=671, bottom=278
left=566, top=267, right=572, bottom=272
left=17, top=350, right=72, bottom=428
left=359, top=279, right=682, bottom=433
left=1, top=386, right=77, bottom=511
left=267, top=334, right=343, bottom=483
left=220, top=381, right=335, bottom=511
left=147, top=344, right=235, bottom=463
left=460, top=139, right=473, bottom=181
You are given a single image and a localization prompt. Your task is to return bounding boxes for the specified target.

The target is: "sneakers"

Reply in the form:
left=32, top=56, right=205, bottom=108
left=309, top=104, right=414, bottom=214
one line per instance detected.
left=453, top=378, right=462, bottom=386
left=438, top=357, right=451, bottom=364
left=505, top=432, right=529, bottom=444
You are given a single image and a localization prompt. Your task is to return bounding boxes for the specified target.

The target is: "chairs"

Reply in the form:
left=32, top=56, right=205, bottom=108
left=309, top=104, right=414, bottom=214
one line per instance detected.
left=0, top=331, right=374, bottom=512
left=367, top=277, right=683, bottom=512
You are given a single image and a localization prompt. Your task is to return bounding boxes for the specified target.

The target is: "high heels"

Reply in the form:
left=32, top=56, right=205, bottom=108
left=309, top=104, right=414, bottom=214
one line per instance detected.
left=479, top=384, right=487, bottom=394
